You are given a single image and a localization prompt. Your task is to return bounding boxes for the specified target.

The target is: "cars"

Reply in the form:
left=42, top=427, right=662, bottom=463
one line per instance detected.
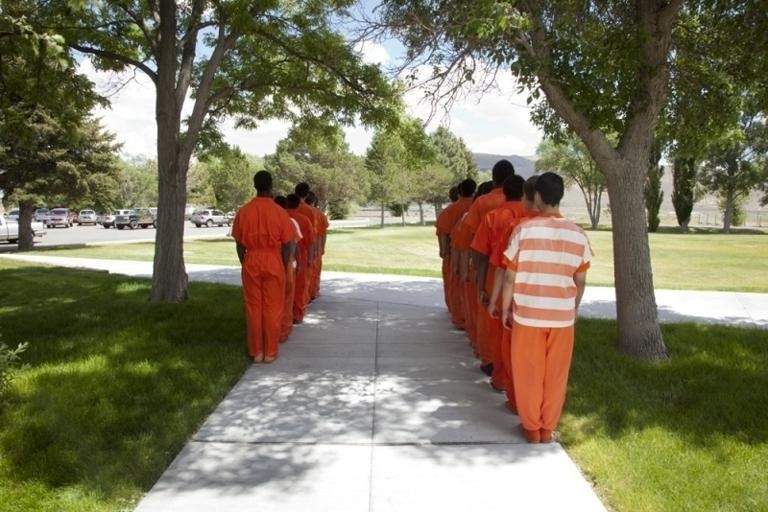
left=0, top=199, right=237, bottom=248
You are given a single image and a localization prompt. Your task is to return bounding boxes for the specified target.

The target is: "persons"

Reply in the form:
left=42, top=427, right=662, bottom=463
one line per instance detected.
left=232, top=169, right=330, bottom=363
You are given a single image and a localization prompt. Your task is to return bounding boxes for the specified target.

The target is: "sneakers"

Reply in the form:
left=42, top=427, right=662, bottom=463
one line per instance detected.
left=480, top=361, right=521, bottom=416
left=540, top=426, right=554, bottom=443
left=518, top=423, right=540, bottom=444
left=253, top=352, right=264, bottom=363
left=263, top=355, right=278, bottom=362
